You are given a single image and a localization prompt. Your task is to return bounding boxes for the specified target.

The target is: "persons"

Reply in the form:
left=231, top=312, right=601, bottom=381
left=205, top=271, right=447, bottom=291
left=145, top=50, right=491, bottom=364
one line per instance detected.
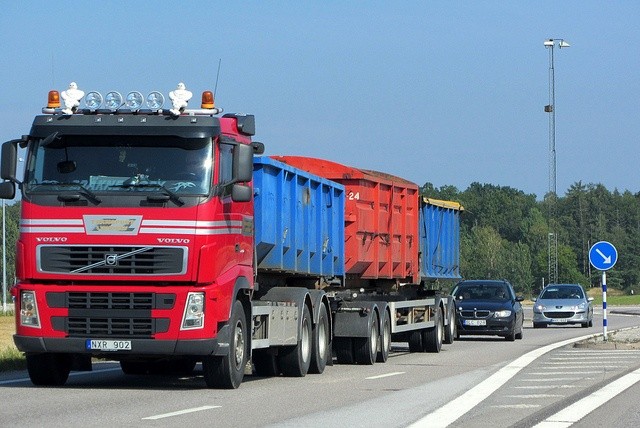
left=568, top=289, right=581, bottom=300
left=550, top=291, right=558, bottom=299
left=495, top=288, right=505, bottom=300
left=463, top=292, right=471, bottom=300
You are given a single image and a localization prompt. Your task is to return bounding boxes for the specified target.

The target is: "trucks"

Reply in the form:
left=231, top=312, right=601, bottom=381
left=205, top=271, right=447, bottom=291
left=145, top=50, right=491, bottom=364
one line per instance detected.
left=1, top=83, right=464, bottom=389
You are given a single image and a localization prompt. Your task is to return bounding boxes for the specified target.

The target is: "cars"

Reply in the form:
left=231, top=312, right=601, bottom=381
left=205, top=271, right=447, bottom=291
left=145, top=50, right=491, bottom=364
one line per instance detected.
left=532, top=284, right=594, bottom=328
left=451, top=280, right=523, bottom=340
